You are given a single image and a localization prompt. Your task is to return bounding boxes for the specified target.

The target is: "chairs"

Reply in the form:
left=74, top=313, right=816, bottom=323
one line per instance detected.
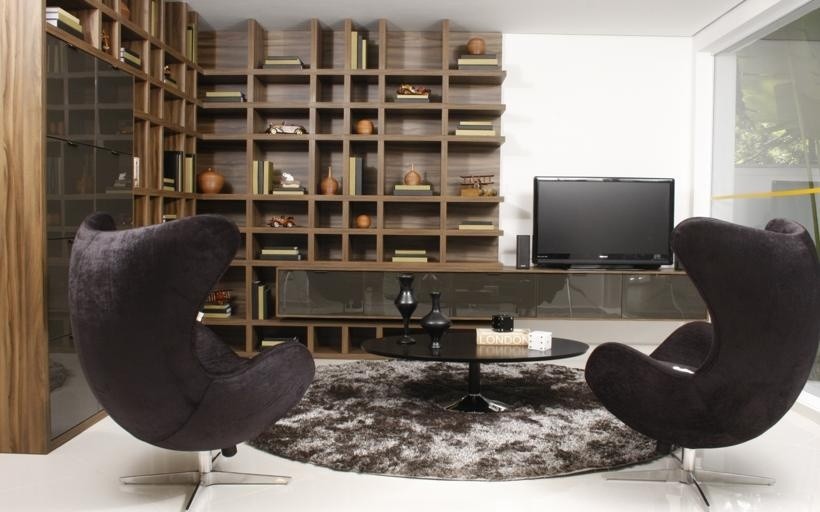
left=69, top=212, right=317, bottom=512
left=583, top=218, right=820, bottom=512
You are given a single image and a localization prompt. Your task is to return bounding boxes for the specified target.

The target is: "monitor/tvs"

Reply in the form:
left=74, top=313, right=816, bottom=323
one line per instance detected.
left=532, top=175, right=675, bottom=268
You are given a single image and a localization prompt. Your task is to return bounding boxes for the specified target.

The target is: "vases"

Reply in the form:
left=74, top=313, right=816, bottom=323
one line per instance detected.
left=419, top=289, right=451, bottom=349
left=393, top=271, right=418, bottom=344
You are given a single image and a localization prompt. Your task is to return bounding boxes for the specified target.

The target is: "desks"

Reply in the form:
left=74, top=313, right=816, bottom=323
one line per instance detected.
left=360, top=328, right=590, bottom=413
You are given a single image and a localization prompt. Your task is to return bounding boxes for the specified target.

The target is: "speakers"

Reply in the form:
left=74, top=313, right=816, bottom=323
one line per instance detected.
left=675, top=255, right=685, bottom=270
left=516, top=234, right=530, bottom=268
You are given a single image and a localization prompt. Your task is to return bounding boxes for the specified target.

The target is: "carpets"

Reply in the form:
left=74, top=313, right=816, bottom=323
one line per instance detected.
left=246, top=359, right=683, bottom=483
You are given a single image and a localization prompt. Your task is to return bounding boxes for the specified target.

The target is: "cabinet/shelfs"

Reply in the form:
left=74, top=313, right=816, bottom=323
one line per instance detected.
left=507, top=265, right=709, bottom=321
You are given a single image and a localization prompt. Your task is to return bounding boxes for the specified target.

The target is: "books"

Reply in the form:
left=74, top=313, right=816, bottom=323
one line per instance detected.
left=201, top=96, right=246, bottom=104
left=349, top=156, right=356, bottom=197
left=396, top=93, right=429, bottom=100
left=350, top=31, right=367, bottom=70
left=454, top=120, right=496, bottom=138
left=393, top=98, right=431, bottom=104
left=355, top=156, right=364, bottom=196
left=118, top=46, right=144, bottom=71
left=395, top=183, right=432, bottom=191
left=392, top=189, right=433, bottom=196
left=163, top=76, right=179, bottom=89
left=258, top=334, right=300, bottom=354
left=161, top=214, right=177, bottom=223
left=391, top=249, right=428, bottom=263
left=200, top=303, right=233, bottom=319
left=456, top=54, right=499, bottom=72
left=462, top=220, right=493, bottom=226
left=251, top=279, right=272, bottom=321
left=258, top=245, right=302, bottom=261
left=252, top=159, right=306, bottom=196
left=205, top=90, right=243, bottom=98
left=261, top=55, right=305, bottom=71
left=45, top=5, right=86, bottom=42
left=186, top=21, right=197, bottom=65
left=458, top=224, right=495, bottom=231
left=162, top=150, right=195, bottom=194
